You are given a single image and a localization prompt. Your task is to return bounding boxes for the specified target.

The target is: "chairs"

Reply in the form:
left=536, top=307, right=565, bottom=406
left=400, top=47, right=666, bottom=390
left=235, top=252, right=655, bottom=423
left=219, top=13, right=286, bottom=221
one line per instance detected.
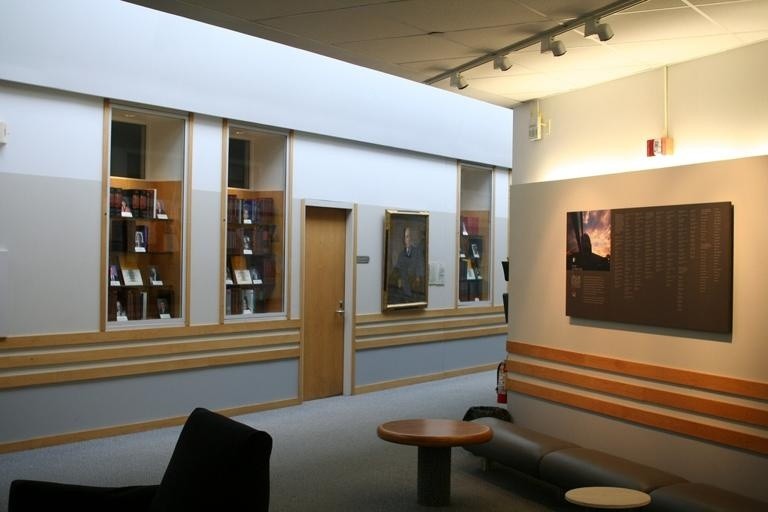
left=7, top=406, right=273, bottom=511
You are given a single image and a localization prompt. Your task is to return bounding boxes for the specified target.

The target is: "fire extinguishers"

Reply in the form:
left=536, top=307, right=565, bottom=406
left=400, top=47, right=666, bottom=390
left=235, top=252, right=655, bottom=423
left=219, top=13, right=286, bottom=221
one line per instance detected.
left=496, top=360, right=507, bottom=405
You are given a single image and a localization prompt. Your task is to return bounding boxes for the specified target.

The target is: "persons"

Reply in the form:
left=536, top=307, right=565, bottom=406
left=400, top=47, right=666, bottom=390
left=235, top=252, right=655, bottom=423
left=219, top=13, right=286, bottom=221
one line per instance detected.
left=393, top=227, right=424, bottom=301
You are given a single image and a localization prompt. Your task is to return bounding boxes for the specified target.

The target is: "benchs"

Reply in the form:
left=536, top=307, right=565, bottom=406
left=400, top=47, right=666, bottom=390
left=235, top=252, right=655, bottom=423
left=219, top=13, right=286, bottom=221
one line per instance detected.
left=460, top=415, right=767, bottom=511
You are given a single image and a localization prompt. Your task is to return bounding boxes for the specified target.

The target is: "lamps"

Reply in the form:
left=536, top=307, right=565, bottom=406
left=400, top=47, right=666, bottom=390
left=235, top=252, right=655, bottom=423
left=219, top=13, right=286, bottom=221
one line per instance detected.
left=449, top=14, right=616, bottom=91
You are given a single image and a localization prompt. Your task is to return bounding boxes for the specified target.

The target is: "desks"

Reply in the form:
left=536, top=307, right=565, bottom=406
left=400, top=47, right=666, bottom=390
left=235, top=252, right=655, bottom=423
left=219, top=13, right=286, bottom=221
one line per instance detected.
left=377, top=419, right=492, bottom=506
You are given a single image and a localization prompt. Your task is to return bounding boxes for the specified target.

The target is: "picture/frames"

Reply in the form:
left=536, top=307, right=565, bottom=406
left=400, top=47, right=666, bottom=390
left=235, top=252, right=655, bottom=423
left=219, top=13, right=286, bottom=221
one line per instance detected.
left=380, top=208, right=430, bottom=313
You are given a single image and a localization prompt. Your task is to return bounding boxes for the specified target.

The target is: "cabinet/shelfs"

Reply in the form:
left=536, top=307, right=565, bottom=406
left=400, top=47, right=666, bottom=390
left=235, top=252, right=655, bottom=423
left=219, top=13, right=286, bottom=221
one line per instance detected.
left=459, top=209, right=490, bottom=302
left=107, top=176, right=183, bottom=324
left=224, top=186, right=284, bottom=316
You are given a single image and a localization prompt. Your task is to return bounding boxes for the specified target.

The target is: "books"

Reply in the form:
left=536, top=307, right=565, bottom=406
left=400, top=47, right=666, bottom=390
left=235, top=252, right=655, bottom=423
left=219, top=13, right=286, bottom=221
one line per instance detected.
left=110, top=186, right=170, bottom=321
left=227, top=199, right=272, bottom=313
left=460, top=220, right=483, bottom=282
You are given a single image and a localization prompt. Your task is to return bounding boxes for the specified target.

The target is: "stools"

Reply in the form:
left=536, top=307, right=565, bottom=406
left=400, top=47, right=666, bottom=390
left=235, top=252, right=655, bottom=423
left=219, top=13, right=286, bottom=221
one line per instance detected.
left=564, top=485, right=651, bottom=510
